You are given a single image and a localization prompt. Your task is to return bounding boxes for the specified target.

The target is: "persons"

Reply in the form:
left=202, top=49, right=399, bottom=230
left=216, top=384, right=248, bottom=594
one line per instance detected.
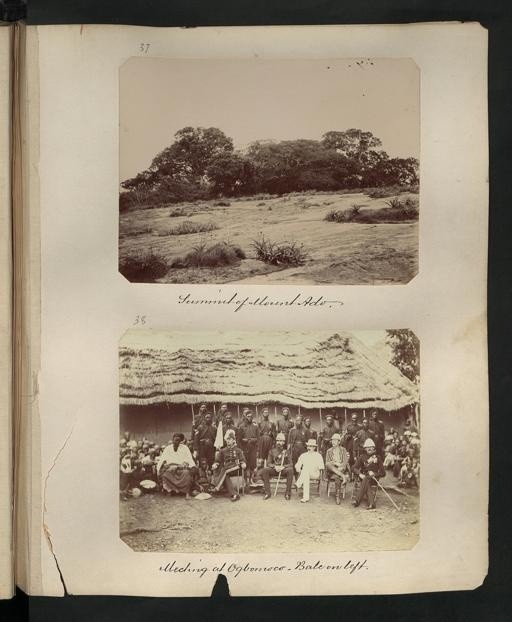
left=120, top=403, right=420, bottom=510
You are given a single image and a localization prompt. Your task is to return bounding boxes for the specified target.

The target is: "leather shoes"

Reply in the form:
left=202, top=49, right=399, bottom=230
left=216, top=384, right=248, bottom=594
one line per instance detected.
left=208, top=485, right=376, bottom=510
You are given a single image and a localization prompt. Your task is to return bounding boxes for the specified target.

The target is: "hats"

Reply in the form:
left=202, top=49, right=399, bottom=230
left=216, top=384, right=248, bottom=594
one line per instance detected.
left=126, top=401, right=419, bottom=451
